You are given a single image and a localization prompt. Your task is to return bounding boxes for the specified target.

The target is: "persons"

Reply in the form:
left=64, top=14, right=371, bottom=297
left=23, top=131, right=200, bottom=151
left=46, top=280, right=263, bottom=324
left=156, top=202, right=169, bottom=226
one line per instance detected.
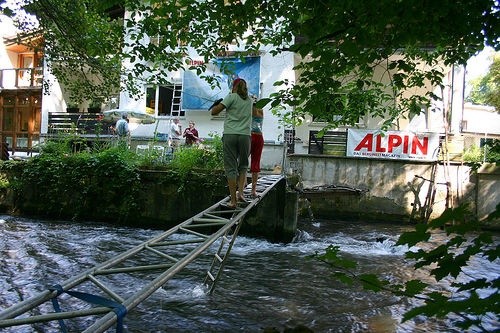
left=247, top=92, right=264, bottom=199
left=115, top=113, right=131, bottom=150
left=211, top=78, right=253, bottom=208
left=168, top=116, right=183, bottom=153
left=183, top=120, right=199, bottom=146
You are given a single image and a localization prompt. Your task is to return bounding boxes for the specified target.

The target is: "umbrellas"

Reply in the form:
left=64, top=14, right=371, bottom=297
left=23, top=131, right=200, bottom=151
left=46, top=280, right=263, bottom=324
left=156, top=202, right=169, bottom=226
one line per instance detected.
left=103, top=108, right=156, bottom=125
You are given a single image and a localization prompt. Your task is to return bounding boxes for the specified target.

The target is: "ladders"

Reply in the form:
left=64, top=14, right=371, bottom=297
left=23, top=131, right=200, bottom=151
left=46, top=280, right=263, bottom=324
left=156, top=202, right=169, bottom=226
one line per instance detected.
left=441, top=107, right=455, bottom=233
left=204, top=203, right=243, bottom=297
left=167, top=84, right=182, bottom=146
